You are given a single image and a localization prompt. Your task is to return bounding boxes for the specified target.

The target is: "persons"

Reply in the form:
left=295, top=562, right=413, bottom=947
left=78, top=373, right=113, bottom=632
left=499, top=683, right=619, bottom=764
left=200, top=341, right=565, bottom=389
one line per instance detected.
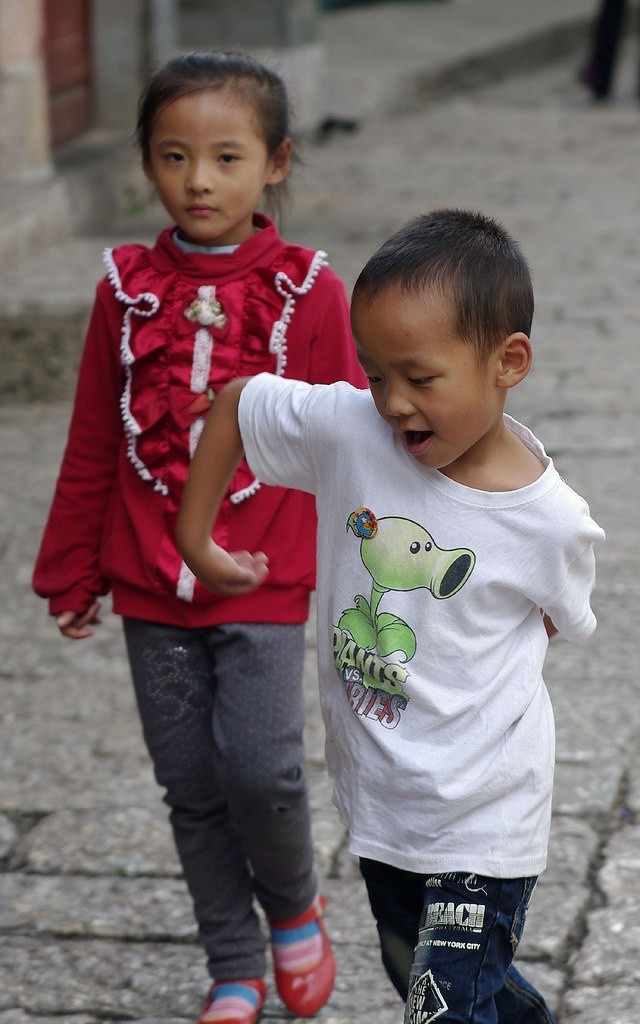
left=27, top=47, right=370, bottom=1024
left=176, top=212, right=607, bottom=1023
left=578, top=0, right=640, bottom=106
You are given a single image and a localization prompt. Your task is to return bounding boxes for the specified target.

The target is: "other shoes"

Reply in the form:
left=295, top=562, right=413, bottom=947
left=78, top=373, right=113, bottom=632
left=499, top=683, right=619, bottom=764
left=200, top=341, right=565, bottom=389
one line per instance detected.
left=198, top=972, right=266, bottom=1024
left=268, top=894, right=335, bottom=1017
